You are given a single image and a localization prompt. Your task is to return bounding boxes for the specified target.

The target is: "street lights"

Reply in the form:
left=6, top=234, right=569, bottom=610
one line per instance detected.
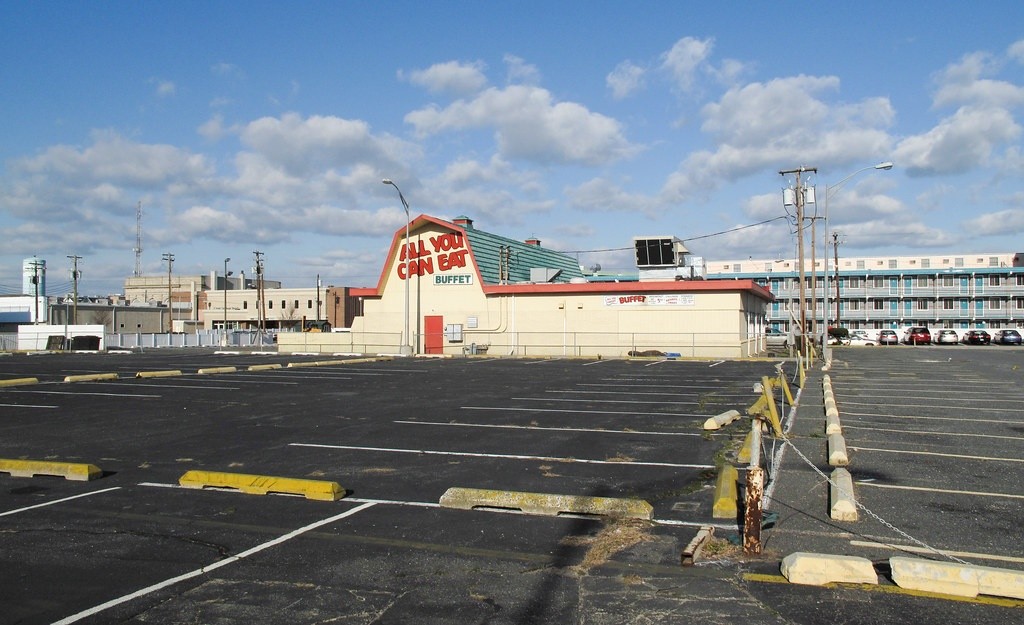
left=381, top=178, right=413, bottom=356
left=222, top=256, right=231, bottom=344
left=821, top=161, right=894, bottom=358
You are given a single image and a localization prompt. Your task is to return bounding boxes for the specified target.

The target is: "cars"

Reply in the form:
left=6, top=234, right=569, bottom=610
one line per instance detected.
left=765, top=326, right=793, bottom=349
left=876, top=330, right=898, bottom=345
left=932, top=329, right=958, bottom=345
left=963, top=330, right=991, bottom=345
left=820, top=329, right=881, bottom=347
left=994, top=329, right=1022, bottom=346
left=902, top=326, right=931, bottom=345
